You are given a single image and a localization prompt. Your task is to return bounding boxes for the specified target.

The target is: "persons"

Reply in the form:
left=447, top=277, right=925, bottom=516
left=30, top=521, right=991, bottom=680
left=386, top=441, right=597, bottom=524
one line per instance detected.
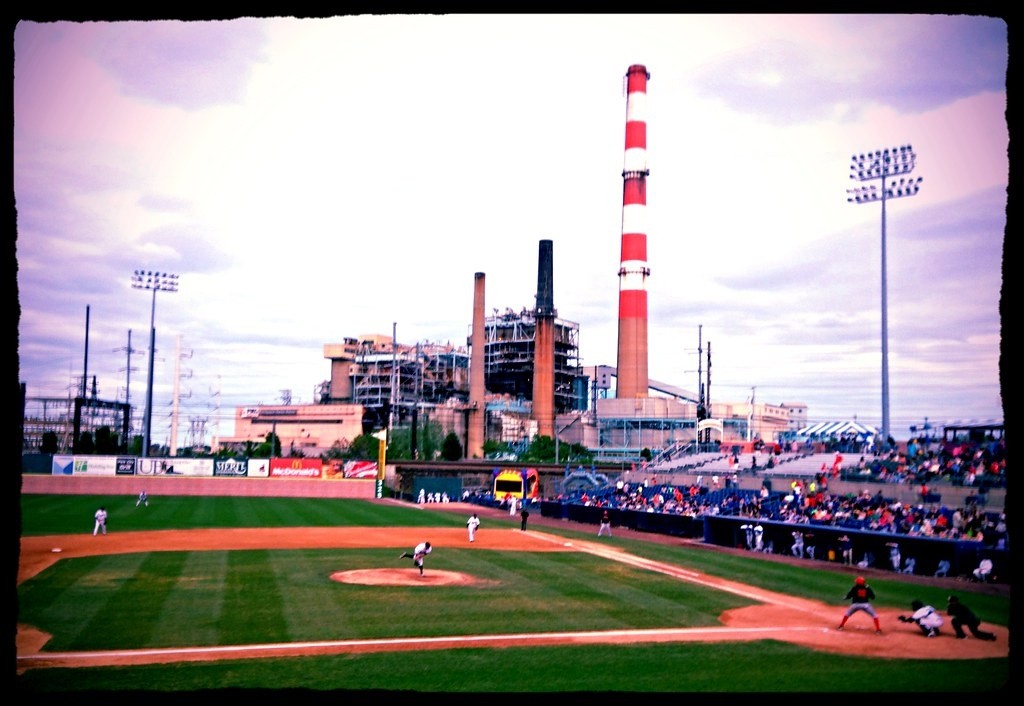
left=579, top=435, right=1005, bottom=582
left=466, top=514, right=480, bottom=542
left=598, top=510, right=612, bottom=537
left=419, top=489, right=450, bottom=503
left=136, top=491, right=148, bottom=506
left=462, top=489, right=517, bottom=516
left=93, top=506, right=107, bottom=536
left=947, top=596, right=997, bottom=641
left=898, top=600, right=943, bottom=637
left=521, top=508, right=529, bottom=531
left=399, top=542, right=433, bottom=577
left=531, top=492, right=571, bottom=508
left=835, top=577, right=881, bottom=635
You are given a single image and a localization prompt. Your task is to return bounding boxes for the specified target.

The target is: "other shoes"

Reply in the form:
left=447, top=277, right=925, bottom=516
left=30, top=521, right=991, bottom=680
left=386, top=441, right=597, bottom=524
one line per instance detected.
left=992, top=633, right=997, bottom=642
left=838, top=625, right=844, bottom=631
left=876, top=629, right=881, bottom=635
left=421, top=572, right=424, bottom=577
left=928, top=630, right=934, bottom=638
left=400, top=552, right=407, bottom=558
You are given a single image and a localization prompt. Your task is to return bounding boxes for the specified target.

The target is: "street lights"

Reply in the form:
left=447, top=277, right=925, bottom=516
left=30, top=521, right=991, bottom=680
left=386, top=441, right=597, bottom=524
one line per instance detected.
left=131, top=269, right=179, bottom=458
left=845, top=145, right=923, bottom=441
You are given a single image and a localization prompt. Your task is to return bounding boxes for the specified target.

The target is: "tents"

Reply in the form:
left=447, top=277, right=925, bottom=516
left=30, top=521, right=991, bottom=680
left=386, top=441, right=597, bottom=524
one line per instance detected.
left=796, top=422, right=882, bottom=439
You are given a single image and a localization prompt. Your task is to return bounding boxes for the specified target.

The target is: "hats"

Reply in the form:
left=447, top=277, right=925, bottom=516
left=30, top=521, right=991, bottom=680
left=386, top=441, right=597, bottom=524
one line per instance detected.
left=855, top=577, right=865, bottom=585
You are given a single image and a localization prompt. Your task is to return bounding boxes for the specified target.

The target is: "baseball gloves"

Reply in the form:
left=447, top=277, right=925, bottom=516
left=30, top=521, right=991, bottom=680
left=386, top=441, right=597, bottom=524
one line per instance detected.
left=897, top=615, right=906, bottom=623
left=103, top=518, right=108, bottom=525
left=414, top=561, right=419, bottom=566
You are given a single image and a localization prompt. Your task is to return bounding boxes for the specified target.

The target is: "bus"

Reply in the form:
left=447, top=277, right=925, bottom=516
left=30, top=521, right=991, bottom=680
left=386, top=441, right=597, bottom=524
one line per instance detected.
left=492, top=467, right=539, bottom=506
